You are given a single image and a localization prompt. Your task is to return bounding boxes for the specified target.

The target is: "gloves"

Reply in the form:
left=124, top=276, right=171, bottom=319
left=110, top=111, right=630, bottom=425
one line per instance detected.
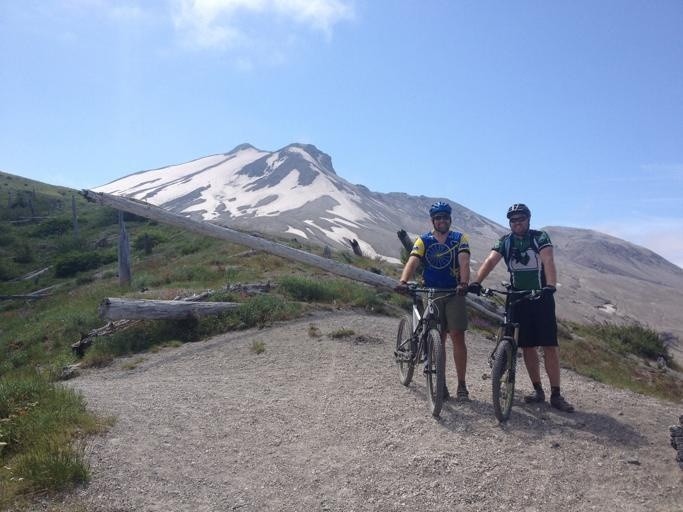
left=397, top=282, right=408, bottom=295
left=543, top=285, right=556, bottom=296
left=456, top=282, right=482, bottom=297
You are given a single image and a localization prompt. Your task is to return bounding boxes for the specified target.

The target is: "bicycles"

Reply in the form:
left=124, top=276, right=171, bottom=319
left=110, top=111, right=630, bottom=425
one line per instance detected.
left=393, top=280, right=551, bottom=422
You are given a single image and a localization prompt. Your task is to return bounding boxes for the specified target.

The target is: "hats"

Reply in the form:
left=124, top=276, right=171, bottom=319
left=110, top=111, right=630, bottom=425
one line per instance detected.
left=429, top=201, right=452, bottom=216
left=507, top=204, right=531, bottom=219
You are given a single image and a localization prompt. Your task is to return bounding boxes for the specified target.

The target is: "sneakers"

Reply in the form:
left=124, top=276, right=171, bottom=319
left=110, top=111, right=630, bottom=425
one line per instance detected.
left=433, top=386, right=450, bottom=401
left=524, top=391, right=545, bottom=402
left=550, top=395, right=574, bottom=412
left=457, top=386, right=469, bottom=401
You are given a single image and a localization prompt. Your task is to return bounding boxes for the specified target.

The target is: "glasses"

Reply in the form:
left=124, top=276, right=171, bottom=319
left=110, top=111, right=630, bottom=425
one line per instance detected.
left=433, top=216, right=450, bottom=220
left=510, top=217, right=529, bottom=223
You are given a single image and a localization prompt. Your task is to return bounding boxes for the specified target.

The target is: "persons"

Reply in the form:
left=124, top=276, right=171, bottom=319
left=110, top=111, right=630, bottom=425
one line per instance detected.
left=396, top=202, right=470, bottom=402
left=468, top=204, right=573, bottom=412
